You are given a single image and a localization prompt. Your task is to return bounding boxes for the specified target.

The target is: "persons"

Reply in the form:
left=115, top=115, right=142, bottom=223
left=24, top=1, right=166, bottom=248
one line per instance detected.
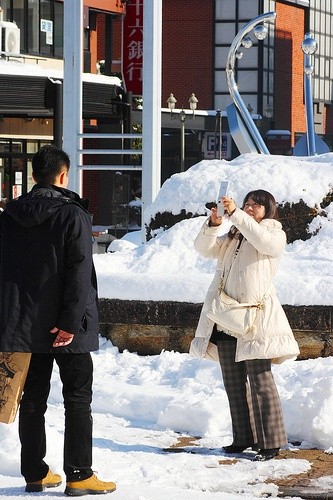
left=189, top=188, right=300, bottom=461
left=0, top=144, right=114, bottom=498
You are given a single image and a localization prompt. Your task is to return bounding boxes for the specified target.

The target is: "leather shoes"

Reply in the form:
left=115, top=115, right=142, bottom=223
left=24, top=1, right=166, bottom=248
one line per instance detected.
left=64, top=471, right=116, bottom=496
left=24, top=470, right=62, bottom=492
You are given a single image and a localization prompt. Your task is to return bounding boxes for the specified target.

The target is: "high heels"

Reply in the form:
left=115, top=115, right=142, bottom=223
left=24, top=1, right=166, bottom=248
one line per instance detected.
left=255, top=447, right=280, bottom=459
left=221, top=443, right=258, bottom=453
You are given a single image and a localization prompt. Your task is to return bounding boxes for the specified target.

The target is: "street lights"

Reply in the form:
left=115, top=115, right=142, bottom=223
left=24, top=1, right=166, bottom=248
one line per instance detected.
left=166, top=92, right=198, bottom=172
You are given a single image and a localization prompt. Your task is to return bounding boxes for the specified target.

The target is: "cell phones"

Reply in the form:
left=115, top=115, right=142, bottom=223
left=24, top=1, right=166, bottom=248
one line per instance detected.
left=216, top=180, right=228, bottom=216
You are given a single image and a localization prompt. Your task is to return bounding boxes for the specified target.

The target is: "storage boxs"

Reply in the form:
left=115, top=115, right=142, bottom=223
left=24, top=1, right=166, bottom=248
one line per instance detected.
left=0, top=352, right=32, bottom=423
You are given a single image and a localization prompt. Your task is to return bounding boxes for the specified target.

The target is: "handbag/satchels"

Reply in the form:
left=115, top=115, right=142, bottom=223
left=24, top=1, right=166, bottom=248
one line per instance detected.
left=206, top=291, right=261, bottom=337
left=0, top=352, right=32, bottom=424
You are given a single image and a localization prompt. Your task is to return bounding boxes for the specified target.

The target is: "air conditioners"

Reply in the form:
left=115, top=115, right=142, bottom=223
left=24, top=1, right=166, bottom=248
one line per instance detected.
left=0, top=28, right=20, bottom=58
